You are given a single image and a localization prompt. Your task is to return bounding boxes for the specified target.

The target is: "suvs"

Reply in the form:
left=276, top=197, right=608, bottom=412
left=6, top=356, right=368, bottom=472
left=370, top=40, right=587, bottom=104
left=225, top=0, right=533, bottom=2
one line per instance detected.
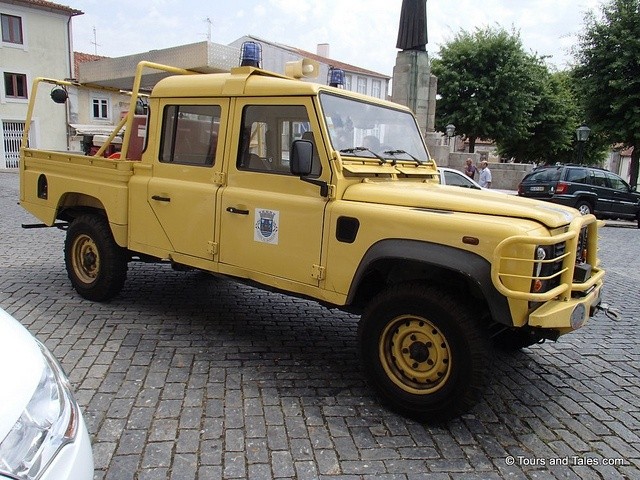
left=516, top=162, right=640, bottom=227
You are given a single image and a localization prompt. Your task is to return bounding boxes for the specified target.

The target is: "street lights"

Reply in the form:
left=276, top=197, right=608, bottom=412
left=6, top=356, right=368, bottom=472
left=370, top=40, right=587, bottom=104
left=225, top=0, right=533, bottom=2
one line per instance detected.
left=444, top=123, right=456, bottom=145
left=576, top=123, right=590, bottom=164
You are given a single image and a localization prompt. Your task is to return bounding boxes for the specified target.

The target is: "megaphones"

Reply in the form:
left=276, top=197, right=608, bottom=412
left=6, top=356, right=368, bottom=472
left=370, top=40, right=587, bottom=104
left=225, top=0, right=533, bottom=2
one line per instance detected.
left=285, top=58, right=321, bottom=80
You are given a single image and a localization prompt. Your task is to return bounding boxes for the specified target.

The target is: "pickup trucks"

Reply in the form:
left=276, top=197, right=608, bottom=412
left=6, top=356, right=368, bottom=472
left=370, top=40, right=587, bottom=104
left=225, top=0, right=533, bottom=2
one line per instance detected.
left=15, top=37, right=620, bottom=427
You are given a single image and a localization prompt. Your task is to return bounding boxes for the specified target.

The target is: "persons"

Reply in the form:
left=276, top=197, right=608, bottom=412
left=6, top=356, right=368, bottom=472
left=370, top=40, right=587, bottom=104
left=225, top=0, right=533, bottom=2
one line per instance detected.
left=362, top=135, right=380, bottom=153
left=464, top=158, right=475, bottom=180
left=473, top=161, right=492, bottom=189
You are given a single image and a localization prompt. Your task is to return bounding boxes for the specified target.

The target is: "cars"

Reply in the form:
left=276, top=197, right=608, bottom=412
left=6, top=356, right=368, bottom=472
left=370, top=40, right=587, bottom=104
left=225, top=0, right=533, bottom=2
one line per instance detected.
left=436, top=166, right=484, bottom=191
left=0, top=307, right=96, bottom=479
